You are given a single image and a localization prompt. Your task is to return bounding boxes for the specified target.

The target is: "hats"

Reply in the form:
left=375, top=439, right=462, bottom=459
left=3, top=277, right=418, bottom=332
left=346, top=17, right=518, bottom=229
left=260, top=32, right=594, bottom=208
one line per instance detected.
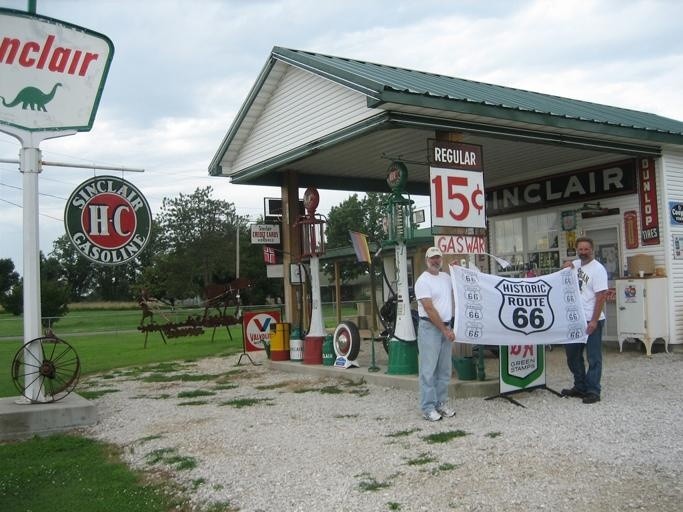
left=425, top=247, right=443, bottom=258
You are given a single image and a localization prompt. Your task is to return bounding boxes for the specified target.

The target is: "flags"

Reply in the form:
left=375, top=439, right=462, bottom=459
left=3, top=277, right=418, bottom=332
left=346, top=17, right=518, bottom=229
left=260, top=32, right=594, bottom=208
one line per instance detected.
left=348, top=230, right=372, bottom=264
left=263, top=247, right=276, bottom=264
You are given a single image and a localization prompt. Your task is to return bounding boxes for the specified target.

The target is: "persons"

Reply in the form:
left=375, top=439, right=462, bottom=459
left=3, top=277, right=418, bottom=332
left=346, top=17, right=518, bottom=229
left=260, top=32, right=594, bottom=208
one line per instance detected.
left=561, top=237, right=609, bottom=403
left=414, top=247, right=457, bottom=422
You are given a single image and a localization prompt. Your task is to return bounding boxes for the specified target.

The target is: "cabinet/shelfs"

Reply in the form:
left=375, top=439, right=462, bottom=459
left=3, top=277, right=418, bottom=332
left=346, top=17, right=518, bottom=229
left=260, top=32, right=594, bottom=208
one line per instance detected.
left=615, top=276, right=670, bottom=356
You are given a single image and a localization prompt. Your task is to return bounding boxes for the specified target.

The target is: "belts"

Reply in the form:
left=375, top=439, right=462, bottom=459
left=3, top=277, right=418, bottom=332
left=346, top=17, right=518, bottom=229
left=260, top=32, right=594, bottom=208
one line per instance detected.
left=421, top=316, right=450, bottom=326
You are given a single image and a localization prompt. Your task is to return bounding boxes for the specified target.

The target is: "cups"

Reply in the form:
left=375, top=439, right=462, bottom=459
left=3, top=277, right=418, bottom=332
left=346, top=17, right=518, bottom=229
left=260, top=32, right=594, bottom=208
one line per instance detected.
left=639, top=270, right=644, bottom=277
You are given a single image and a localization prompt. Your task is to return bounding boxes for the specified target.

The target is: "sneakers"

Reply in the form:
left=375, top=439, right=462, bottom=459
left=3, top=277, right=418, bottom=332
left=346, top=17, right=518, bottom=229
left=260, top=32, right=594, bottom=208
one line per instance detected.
left=422, top=409, right=443, bottom=422
left=582, top=393, right=600, bottom=403
left=435, top=403, right=456, bottom=418
left=561, top=388, right=585, bottom=398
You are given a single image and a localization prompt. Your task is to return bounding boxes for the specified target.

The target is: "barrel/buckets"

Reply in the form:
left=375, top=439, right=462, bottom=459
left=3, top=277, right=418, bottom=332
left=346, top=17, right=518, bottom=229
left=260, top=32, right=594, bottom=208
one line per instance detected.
left=289, top=327, right=304, bottom=361
left=269, top=322, right=290, bottom=361
left=320, top=332, right=336, bottom=366
